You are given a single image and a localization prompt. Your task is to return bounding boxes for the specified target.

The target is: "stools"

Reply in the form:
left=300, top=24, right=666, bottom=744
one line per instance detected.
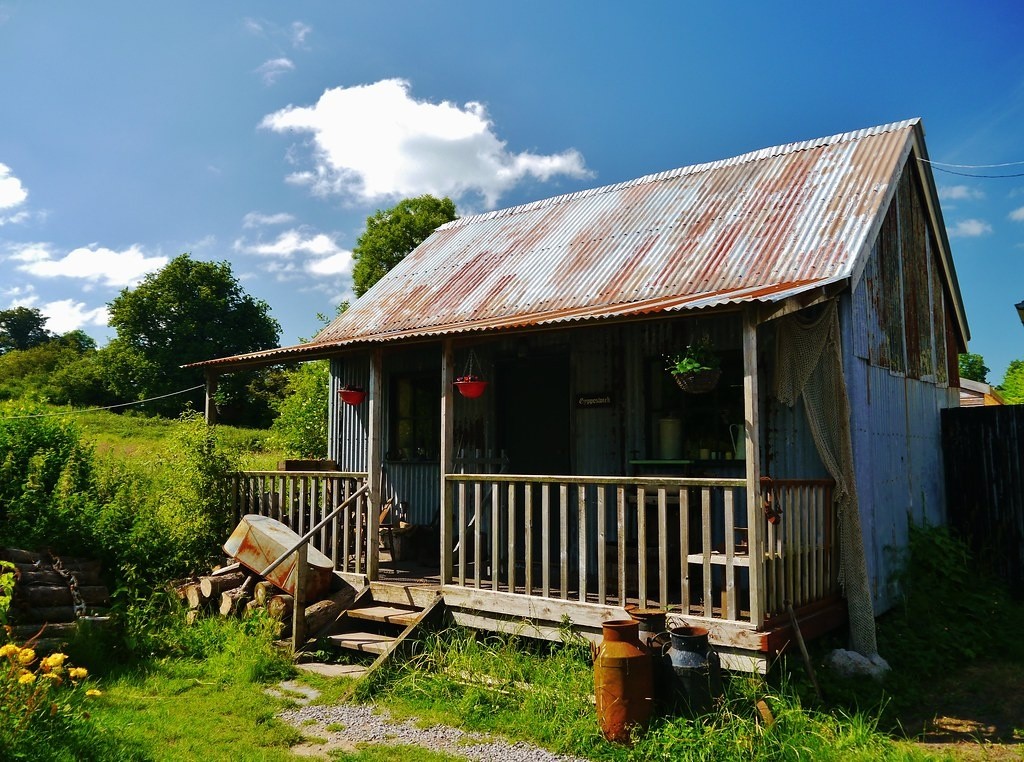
left=348, top=524, right=397, bottom=574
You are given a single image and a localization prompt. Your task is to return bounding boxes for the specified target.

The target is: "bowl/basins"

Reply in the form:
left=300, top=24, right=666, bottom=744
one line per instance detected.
left=453, top=382, right=489, bottom=397
left=338, top=390, right=368, bottom=405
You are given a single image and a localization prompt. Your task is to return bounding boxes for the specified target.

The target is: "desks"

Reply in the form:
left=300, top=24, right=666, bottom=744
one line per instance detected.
left=628, top=459, right=745, bottom=555
left=686, top=550, right=777, bottom=620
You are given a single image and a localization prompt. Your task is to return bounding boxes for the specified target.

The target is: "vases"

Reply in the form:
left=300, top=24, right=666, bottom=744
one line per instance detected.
left=337, top=389, right=367, bottom=404
left=453, top=381, right=489, bottom=399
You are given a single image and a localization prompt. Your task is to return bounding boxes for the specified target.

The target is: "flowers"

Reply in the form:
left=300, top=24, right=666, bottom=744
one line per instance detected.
left=340, top=384, right=364, bottom=391
left=455, top=374, right=479, bottom=382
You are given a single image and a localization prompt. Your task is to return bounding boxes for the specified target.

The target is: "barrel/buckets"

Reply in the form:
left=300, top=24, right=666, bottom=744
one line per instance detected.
left=656, top=417, right=683, bottom=459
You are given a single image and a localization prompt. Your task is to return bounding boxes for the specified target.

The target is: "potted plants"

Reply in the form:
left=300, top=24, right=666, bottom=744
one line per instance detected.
left=663, top=333, right=723, bottom=391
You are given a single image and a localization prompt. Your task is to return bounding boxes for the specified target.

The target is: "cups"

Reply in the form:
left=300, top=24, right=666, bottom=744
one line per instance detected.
left=718, top=452, right=724, bottom=459
left=711, top=452, right=716, bottom=459
left=699, top=449, right=709, bottom=459
left=726, top=453, right=732, bottom=460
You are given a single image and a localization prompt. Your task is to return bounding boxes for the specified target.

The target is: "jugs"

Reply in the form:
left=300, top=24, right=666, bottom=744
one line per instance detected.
left=730, top=424, right=745, bottom=460
